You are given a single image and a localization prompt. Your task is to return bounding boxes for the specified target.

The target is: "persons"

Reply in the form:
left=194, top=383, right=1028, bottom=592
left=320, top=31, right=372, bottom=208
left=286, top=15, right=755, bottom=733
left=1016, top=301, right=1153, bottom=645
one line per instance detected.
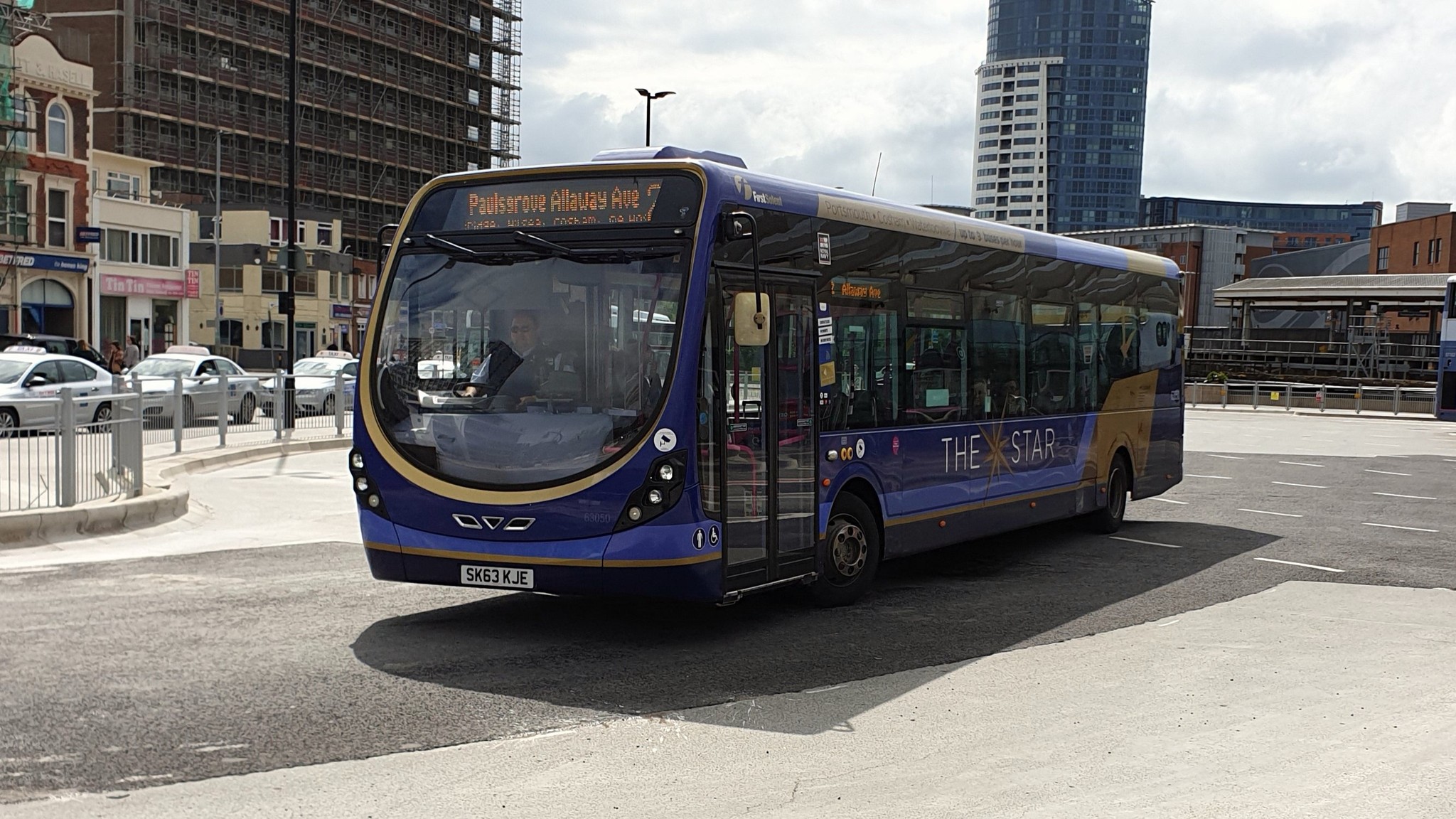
left=973, top=378, right=987, bottom=421
left=124, top=336, right=139, bottom=369
left=345, top=341, right=351, bottom=352
left=1001, top=378, right=1035, bottom=418
left=461, top=310, right=552, bottom=397
left=73, top=340, right=97, bottom=363
left=108, top=340, right=124, bottom=375
left=556, top=304, right=646, bottom=429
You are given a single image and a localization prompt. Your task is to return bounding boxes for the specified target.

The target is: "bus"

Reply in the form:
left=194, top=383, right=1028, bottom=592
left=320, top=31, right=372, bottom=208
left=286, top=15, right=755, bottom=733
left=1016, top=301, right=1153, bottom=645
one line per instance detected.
left=609, top=304, right=675, bottom=389
left=345, top=145, right=1185, bottom=611
left=1434, top=275, right=1456, bottom=422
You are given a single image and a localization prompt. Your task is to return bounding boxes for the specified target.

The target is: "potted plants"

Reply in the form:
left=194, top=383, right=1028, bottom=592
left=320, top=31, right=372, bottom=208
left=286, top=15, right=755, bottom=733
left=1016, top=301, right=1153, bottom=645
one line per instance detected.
left=1200, top=372, right=1229, bottom=401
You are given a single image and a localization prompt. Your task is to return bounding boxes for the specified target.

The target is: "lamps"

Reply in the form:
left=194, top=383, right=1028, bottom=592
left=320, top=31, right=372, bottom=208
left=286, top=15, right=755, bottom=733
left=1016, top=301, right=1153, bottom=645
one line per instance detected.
left=246, top=324, right=250, bottom=330
left=200, top=323, right=203, bottom=330
left=1415, top=318, right=1420, bottom=322
left=323, top=327, right=326, bottom=333
left=1409, top=317, right=1413, bottom=321
left=255, top=325, right=259, bottom=331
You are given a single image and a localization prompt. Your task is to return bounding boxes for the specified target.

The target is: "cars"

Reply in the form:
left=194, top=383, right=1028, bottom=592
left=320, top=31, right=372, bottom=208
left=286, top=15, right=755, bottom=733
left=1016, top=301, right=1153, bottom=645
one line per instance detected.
left=256, top=350, right=360, bottom=418
left=121, top=345, right=262, bottom=428
left=0, top=345, right=129, bottom=440
left=417, top=354, right=469, bottom=405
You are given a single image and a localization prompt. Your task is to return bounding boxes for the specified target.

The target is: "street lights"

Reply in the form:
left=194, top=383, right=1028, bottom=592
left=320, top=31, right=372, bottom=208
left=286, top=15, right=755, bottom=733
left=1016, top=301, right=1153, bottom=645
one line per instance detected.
left=635, top=87, right=676, bottom=146
